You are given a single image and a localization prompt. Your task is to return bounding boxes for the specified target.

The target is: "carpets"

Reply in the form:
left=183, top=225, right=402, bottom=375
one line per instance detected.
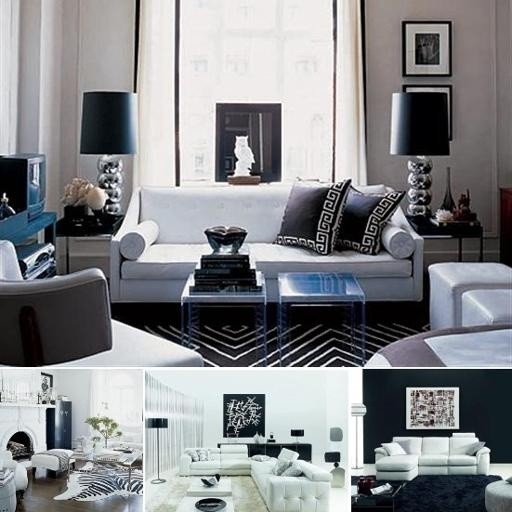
left=53, top=461, right=143, bottom=502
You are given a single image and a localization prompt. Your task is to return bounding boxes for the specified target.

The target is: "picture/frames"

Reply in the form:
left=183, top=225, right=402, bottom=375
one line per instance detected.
left=402, top=22, right=452, bottom=77
left=41, top=372, right=53, bottom=401
left=403, top=85, right=451, bottom=141
left=405, top=386, right=459, bottom=430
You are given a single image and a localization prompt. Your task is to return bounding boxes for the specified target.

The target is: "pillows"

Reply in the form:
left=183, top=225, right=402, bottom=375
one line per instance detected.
left=252, top=454, right=271, bottom=462
left=280, top=464, right=302, bottom=476
left=381, top=437, right=486, bottom=456
left=120, top=177, right=414, bottom=261
left=185, top=448, right=200, bottom=462
left=272, top=458, right=290, bottom=476
left=196, top=449, right=208, bottom=461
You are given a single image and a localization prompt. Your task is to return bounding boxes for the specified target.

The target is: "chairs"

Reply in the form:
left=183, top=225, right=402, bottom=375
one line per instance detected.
left=0, top=239, right=204, bottom=367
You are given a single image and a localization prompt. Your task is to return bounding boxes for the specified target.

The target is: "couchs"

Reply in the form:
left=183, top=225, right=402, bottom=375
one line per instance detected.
left=180, top=445, right=251, bottom=477
left=248, top=447, right=333, bottom=512
left=110, top=187, right=423, bottom=303
left=374, top=447, right=490, bottom=482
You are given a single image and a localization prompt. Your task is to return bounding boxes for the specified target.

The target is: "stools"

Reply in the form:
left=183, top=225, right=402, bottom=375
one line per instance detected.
left=428, top=263, right=512, bottom=331
left=31, top=449, right=73, bottom=480
left=461, top=289, right=512, bottom=327
left=485, top=480, right=512, bottom=512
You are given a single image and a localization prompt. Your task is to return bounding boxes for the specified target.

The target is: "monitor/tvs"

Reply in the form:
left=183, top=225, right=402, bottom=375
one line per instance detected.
left=0, top=154, right=46, bottom=222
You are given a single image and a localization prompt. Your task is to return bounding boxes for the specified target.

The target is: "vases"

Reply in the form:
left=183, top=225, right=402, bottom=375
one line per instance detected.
left=440, top=167, right=456, bottom=213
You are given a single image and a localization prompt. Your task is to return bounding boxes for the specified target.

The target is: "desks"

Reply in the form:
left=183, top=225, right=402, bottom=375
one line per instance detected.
left=405, top=215, right=483, bottom=262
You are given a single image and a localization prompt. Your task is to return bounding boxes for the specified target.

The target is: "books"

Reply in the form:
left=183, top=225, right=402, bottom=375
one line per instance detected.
left=93, top=447, right=135, bottom=464
left=370, top=482, right=394, bottom=495
left=430, top=216, right=468, bottom=227
left=189, top=245, right=266, bottom=296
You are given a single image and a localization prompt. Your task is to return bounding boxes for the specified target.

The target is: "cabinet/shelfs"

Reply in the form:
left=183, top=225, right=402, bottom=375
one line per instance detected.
left=5, top=213, right=57, bottom=279
left=46, top=401, right=71, bottom=479
left=46, top=217, right=124, bottom=279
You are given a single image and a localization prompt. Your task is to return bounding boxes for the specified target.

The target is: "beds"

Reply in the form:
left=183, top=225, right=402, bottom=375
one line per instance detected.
left=75, top=448, right=143, bottom=496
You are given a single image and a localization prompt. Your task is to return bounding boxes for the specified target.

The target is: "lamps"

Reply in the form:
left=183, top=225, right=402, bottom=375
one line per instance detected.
left=79, top=92, right=139, bottom=215
left=291, top=430, right=304, bottom=451
left=350, top=403, right=367, bottom=469
left=145, top=418, right=168, bottom=484
left=389, top=93, right=450, bottom=217
